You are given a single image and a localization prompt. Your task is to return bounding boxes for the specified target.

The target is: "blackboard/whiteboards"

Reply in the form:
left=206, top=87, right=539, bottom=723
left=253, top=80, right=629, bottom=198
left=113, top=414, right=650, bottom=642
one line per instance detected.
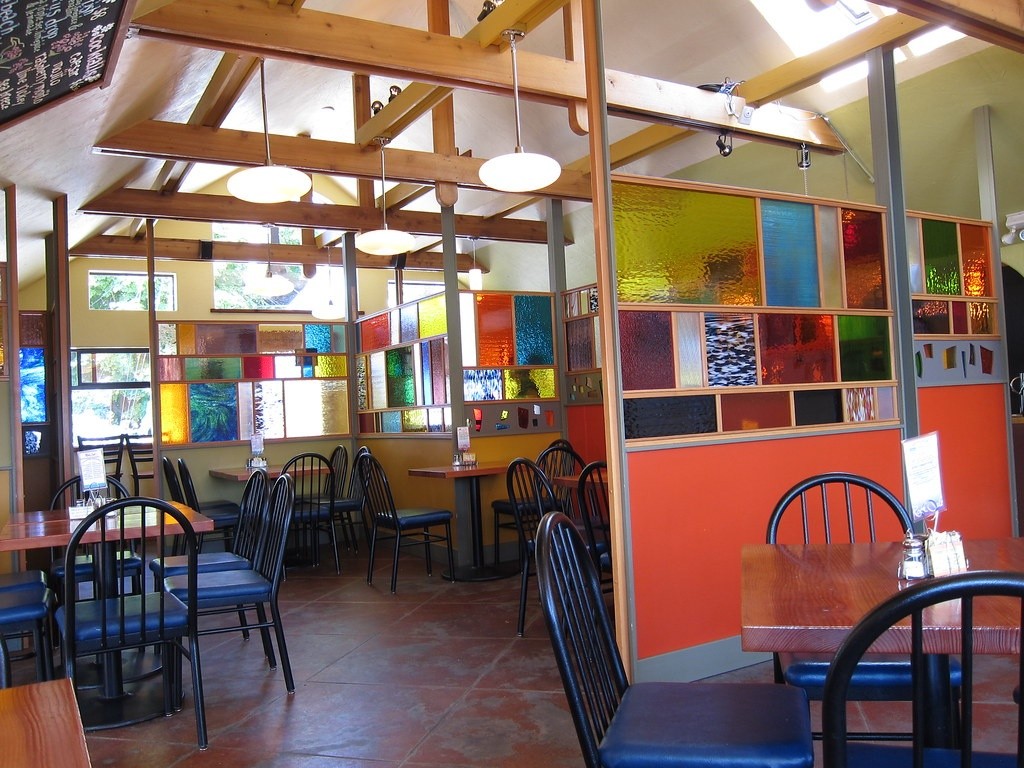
left=0, top=0, right=138, bottom=129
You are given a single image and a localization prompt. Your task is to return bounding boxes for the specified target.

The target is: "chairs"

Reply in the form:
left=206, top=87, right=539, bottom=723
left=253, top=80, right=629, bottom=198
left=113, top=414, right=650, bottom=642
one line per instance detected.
left=492, top=438, right=1024, bottom=768
left=0, top=445, right=458, bottom=751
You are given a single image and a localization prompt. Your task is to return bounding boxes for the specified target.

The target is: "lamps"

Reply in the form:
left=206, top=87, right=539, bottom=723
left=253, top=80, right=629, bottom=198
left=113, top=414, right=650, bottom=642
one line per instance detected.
left=795, top=148, right=811, bottom=171
left=477, top=29, right=561, bottom=192
left=388, top=85, right=401, bottom=101
left=372, top=100, right=385, bottom=115
left=355, top=137, right=417, bottom=256
left=468, top=237, right=483, bottom=290
left=310, top=252, right=345, bottom=320
left=1001, top=210, right=1024, bottom=245
left=394, top=252, right=408, bottom=272
left=243, top=223, right=294, bottom=296
left=227, top=59, right=312, bottom=204
left=477, top=0, right=505, bottom=21
left=198, top=240, right=213, bottom=259
left=715, top=131, right=733, bottom=158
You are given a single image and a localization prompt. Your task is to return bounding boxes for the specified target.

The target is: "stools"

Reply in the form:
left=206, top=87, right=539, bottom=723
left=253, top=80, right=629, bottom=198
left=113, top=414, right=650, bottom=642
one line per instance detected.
left=125, top=433, right=152, bottom=496
left=77, top=432, right=125, bottom=500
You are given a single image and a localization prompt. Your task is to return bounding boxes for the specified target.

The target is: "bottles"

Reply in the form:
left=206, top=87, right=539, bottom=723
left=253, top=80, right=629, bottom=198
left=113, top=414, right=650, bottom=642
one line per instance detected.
left=75, top=499, right=83, bottom=507
left=453, top=455, right=460, bottom=466
left=247, top=458, right=252, bottom=467
left=902, top=538, right=928, bottom=579
left=262, top=458, right=267, bottom=467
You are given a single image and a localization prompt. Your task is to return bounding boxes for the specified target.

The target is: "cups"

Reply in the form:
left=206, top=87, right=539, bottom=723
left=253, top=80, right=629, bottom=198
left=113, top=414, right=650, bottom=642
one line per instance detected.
left=93, top=498, right=106, bottom=510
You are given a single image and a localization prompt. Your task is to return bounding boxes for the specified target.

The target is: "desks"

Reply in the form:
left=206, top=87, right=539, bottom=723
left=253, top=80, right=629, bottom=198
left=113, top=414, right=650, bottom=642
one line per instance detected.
left=4, top=500, right=193, bottom=689
left=551, top=472, right=609, bottom=492
left=740, top=537, right=1024, bottom=752
left=209, top=464, right=336, bottom=567
left=0, top=506, right=215, bottom=732
left=0, top=676, right=93, bottom=768
left=408, top=462, right=549, bottom=583
